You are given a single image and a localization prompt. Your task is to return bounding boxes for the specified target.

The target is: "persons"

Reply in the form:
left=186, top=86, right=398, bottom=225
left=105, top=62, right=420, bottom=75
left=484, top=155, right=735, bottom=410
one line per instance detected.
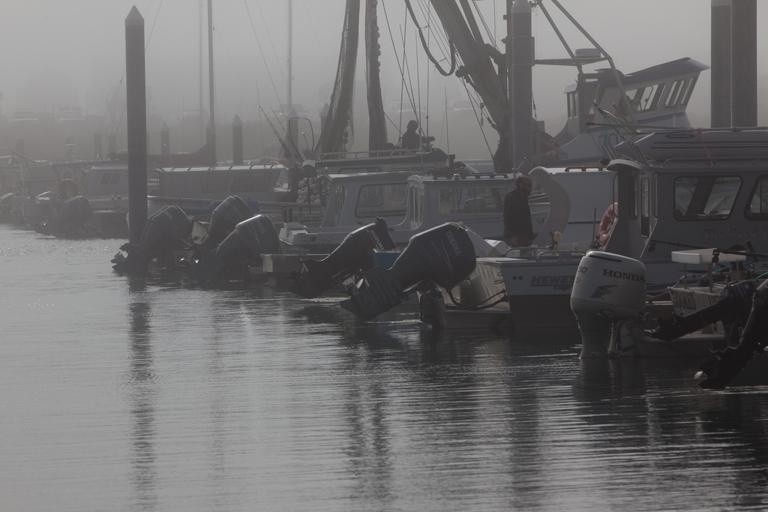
left=402, top=118, right=423, bottom=149
left=504, top=176, right=536, bottom=248
